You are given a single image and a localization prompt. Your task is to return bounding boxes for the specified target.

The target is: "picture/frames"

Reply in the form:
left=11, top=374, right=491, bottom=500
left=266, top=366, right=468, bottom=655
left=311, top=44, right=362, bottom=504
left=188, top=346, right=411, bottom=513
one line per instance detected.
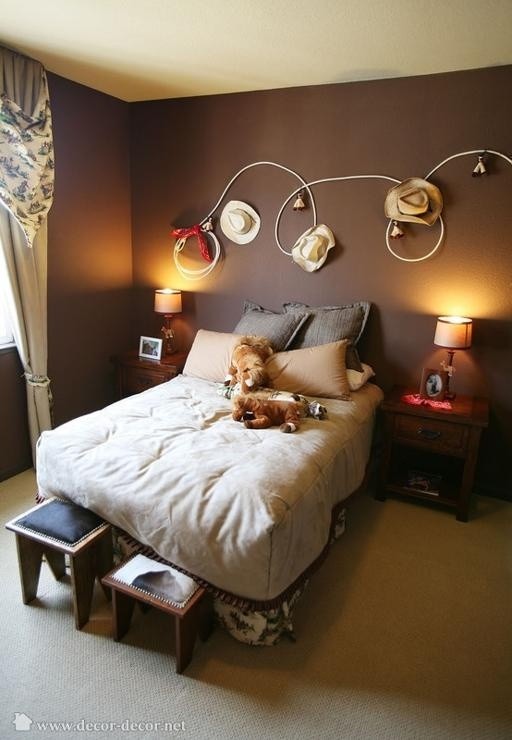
left=419, top=368, right=448, bottom=401
left=139, top=337, right=162, bottom=360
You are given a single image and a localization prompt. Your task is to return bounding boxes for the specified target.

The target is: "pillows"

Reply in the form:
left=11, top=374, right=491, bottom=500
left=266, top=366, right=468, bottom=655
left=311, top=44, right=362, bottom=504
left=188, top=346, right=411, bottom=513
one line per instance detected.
left=184, top=328, right=270, bottom=384
left=347, top=363, right=374, bottom=390
left=264, top=339, right=352, bottom=402
left=281, top=300, right=371, bottom=373
left=230, top=299, right=311, bottom=353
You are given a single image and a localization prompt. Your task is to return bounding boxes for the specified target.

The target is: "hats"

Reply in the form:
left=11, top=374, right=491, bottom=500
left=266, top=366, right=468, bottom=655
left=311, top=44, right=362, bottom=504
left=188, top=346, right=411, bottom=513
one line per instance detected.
left=291, top=223, right=336, bottom=272
left=220, top=200, right=261, bottom=246
left=384, top=176, right=444, bottom=227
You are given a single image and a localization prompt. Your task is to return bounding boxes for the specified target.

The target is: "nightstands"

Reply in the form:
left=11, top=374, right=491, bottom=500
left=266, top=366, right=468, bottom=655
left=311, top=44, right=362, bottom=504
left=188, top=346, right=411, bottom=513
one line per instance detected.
left=112, top=348, right=186, bottom=400
left=379, top=385, right=490, bottom=522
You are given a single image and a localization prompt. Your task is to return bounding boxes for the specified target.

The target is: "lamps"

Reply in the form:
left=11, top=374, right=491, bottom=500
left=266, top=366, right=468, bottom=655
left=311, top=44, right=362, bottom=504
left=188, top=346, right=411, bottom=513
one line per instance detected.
left=154, top=290, right=182, bottom=354
left=433, top=317, right=472, bottom=400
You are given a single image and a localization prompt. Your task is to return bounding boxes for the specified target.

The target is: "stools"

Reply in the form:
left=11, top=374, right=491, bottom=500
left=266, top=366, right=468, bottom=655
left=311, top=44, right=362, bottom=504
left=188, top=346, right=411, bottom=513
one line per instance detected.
left=101, top=552, right=214, bottom=672
left=4, top=496, right=115, bottom=629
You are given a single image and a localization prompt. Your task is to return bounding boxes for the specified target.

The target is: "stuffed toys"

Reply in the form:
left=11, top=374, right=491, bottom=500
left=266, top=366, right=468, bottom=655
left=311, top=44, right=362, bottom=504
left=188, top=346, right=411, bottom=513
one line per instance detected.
left=231, top=390, right=328, bottom=433
left=217, top=334, right=274, bottom=398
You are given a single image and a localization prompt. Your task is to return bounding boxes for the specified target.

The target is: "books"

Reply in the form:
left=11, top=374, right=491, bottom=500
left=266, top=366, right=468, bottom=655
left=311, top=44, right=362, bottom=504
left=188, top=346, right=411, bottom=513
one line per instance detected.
left=401, top=465, right=444, bottom=496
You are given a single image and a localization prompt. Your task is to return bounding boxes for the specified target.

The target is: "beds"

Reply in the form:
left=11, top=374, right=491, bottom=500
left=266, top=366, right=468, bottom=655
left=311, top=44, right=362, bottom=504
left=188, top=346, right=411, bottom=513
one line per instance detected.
left=37, top=302, right=387, bottom=647
